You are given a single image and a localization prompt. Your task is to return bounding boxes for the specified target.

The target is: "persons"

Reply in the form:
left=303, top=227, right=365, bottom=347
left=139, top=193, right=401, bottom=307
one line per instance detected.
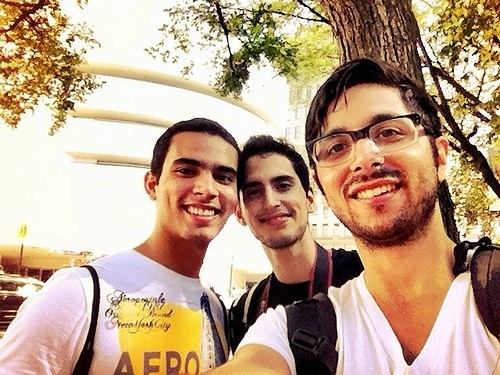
left=0, top=117, right=242, bottom=375
left=202, top=56, right=500, bottom=375
left=228, top=135, right=364, bottom=356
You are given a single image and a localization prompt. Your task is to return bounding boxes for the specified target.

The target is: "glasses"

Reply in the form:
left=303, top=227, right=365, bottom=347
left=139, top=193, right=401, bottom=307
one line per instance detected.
left=305, top=113, right=436, bottom=168
